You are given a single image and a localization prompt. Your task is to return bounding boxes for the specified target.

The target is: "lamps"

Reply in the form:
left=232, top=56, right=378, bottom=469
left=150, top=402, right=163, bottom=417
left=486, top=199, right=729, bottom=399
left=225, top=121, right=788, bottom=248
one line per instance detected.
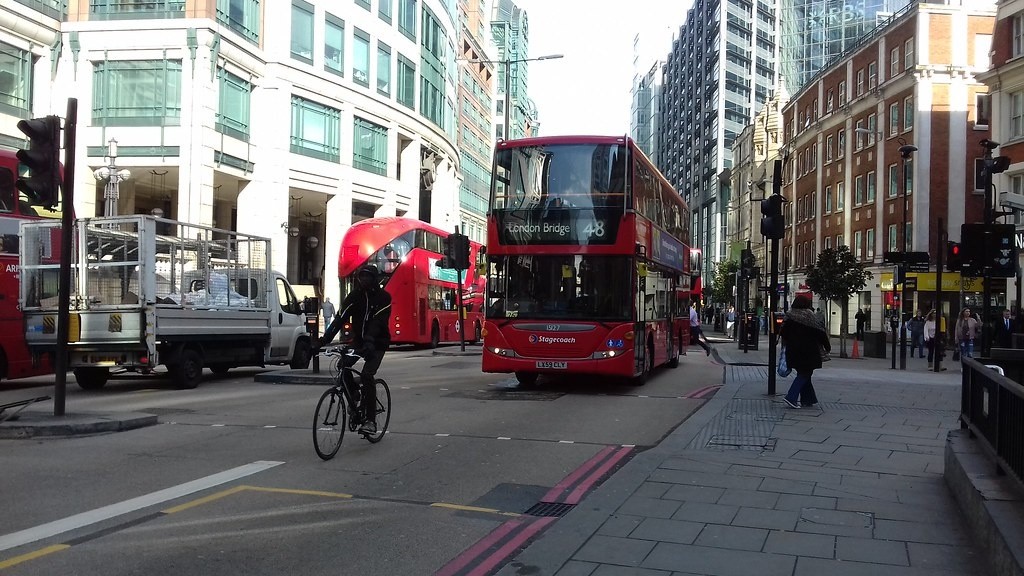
left=855, top=128, right=883, bottom=141
left=212, top=184, right=223, bottom=228
left=763, top=178, right=784, bottom=187
left=147, top=168, right=169, bottom=218
left=289, top=195, right=304, bottom=238
left=302, top=211, right=323, bottom=249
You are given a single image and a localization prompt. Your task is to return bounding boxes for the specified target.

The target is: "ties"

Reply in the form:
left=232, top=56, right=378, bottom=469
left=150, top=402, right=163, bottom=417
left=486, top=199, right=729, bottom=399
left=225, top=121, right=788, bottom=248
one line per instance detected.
left=1006, top=319, right=1008, bottom=329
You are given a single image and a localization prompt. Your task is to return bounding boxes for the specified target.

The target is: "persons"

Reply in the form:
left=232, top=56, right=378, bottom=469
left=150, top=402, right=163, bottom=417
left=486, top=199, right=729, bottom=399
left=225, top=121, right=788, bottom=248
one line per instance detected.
left=690, top=299, right=711, bottom=357
left=955, top=308, right=981, bottom=373
left=321, top=297, right=335, bottom=331
left=993, top=309, right=1014, bottom=348
left=924, top=309, right=948, bottom=371
left=814, top=307, right=825, bottom=324
left=855, top=308, right=866, bottom=341
left=307, top=265, right=391, bottom=435
left=907, top=309, right=926, bottom=358
left=704, top=304, right=735, bottom=339
left=779, top=295, right=831, bottom=408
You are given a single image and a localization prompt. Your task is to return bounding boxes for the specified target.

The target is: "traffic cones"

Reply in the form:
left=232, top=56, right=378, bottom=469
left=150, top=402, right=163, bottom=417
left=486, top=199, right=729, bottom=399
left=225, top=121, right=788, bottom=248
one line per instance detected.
left=850, top=337, right=861, bottom=358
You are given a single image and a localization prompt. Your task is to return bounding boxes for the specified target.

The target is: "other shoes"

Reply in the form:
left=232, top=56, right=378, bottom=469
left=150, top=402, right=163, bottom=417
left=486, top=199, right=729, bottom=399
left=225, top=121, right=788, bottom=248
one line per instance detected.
left=920, top=355, right=926, bottom=358
left=347, top=392, right=360, bottom=415
left=706, top=347, right=710, bottom=356
left=783, top=396, right=802, bottom=409
left=801, top=401, right=813, bottom=407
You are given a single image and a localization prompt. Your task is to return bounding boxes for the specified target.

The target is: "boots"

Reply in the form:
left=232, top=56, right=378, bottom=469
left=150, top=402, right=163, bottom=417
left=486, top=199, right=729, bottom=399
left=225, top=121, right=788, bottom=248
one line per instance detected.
left=927, top=362, right=933, bottom=371
left=939, top=361, right=947, bottom=371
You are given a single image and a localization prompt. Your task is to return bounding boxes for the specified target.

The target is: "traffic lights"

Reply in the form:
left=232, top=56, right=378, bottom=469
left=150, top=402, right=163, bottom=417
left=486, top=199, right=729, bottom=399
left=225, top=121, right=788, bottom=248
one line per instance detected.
left=740, top=248, right=757, bottom=279
left=945, top=240, right=962, bottom=270
left=759, top=197, right=786, bottom=242
left=13, top=113, right=60, bottom=210
left=440, top=233, right=472, bottom=270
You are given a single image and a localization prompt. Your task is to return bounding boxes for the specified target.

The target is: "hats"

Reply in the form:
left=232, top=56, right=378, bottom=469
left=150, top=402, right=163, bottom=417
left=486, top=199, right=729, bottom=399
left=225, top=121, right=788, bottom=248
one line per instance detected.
left=359, top=266, right=378, bottom=281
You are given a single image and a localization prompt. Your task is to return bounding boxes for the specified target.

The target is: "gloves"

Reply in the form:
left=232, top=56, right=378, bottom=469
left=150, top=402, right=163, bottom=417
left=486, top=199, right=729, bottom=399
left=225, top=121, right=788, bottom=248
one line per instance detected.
left=311, top=336, right=327, bottom=355
left=337, top=344, right=349, bottom=353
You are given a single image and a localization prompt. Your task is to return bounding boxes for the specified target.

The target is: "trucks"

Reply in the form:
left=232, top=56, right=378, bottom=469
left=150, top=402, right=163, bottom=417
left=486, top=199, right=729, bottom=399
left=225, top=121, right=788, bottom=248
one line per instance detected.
left=16, top=211, right=313, bottom=390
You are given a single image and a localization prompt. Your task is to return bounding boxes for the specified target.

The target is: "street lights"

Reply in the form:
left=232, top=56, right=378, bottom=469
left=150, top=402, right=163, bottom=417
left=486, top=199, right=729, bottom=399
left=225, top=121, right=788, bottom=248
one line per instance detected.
left=979, top=137, right=1012, bottom=359
left=894, top=144, right=920, bottom=369
left=468, top=54, right=566, bottom=209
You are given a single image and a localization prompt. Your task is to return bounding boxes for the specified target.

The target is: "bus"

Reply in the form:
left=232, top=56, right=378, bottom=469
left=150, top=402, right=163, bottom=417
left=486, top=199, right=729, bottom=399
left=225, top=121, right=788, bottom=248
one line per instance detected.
left=336, top=216, right=489, bottom=348
left=0, top=147, right=80, bottom=385
left=687, top=247, right=703, bottom=344
left=479, top=133, right=693, bottom=388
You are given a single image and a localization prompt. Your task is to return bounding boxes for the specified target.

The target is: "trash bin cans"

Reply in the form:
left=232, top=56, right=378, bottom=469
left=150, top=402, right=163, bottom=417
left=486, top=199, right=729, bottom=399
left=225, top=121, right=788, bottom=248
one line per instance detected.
left=863, top=329, right=887, bottom=359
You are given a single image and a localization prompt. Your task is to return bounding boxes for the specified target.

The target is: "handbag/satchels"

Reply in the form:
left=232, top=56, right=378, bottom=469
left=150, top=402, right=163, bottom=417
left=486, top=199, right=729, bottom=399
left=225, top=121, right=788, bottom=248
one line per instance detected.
left=777, top=347, right=792, bottom=377
left=953, top=343, right=960, bottom=361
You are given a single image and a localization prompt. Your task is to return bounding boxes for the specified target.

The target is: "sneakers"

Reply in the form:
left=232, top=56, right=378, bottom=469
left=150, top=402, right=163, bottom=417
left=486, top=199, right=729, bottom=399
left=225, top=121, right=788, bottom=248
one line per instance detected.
left=358, top=420, right=376, bottom=435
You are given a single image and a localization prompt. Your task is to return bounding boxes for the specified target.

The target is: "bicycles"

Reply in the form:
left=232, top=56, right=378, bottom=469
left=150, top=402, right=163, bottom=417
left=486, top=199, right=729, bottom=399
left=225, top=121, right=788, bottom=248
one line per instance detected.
left=310, top=340, right=394, bottom=460
